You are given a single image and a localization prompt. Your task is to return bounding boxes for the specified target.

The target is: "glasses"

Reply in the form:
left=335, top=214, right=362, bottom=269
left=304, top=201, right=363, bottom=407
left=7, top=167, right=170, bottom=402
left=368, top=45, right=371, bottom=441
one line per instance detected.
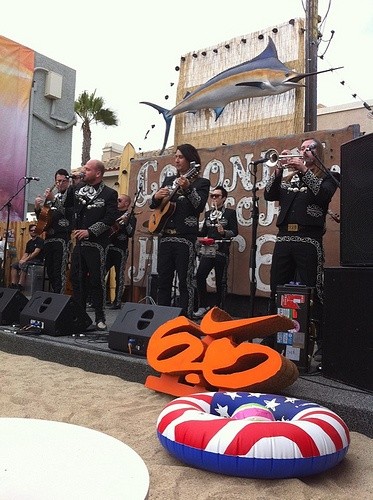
left=210, top=193, right=222, bottom=199
left=118, top=198, right=126, bottom=204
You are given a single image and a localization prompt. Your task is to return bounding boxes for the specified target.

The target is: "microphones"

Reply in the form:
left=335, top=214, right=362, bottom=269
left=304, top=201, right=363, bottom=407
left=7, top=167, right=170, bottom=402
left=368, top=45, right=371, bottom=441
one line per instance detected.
left=23, top=177, right=40, bottom=181
left=65, top=173, right=84, bottom=179
left=249, top=155, right=272, bottom=165
left=306, top=142, right=317, bottom=151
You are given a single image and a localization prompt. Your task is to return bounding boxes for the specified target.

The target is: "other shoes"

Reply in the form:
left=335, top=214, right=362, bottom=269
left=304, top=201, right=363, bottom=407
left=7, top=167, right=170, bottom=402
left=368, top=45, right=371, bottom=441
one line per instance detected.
left=192, top=307, right=210, bottom=318
left=8, top=282, right=17, bottom=289
left=17, top=284, right=25, bottom=291
left=109, top=302, right=121, bottom=310
left=96, top=322, right=108, bottom=331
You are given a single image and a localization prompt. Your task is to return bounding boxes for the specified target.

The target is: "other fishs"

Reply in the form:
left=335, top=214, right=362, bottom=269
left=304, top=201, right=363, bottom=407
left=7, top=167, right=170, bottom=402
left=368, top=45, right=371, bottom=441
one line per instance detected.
left=135, top=35, right=348, bottom=159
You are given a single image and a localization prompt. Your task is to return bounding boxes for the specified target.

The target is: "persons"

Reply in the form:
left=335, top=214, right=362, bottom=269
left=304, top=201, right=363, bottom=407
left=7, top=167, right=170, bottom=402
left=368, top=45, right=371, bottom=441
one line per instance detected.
left=105, top=193, right=137, bottom=310
left=264, top=138, right=341, bottom=351
left=147, top=144, right=210, bottom=322
left=193, top=185, right=239, bottom=319
left=63, top=159, right=118, bottom=329
left=8, top=224, right=45, bottom=290
left=33, top=168, right=73, bottom=293
left=0, top=236, right=9, bottom=283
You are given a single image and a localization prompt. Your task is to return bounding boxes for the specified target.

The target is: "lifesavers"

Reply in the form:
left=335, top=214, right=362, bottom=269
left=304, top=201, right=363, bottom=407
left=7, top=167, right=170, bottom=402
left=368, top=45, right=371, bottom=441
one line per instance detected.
left=156, top=391, right=350, bottom=480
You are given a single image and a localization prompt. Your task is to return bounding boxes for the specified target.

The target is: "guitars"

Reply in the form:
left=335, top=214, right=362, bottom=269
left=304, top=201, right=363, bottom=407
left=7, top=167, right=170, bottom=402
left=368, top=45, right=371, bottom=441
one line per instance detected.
left=147, top=164, right=200, bottom=236
left=108, top=211, right=131, bottom=243
left=33, top=181, right=59, bottom=236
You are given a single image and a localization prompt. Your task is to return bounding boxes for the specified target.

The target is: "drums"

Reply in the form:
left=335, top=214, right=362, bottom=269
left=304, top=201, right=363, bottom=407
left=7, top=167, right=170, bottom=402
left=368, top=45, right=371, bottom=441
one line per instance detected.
left=196, top=244, right=218, bottom=258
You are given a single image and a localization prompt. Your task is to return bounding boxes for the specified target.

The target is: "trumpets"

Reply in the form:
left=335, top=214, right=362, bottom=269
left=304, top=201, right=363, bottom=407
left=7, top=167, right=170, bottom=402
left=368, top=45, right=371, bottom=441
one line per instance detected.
left=265, top=149, right=307, bottom=169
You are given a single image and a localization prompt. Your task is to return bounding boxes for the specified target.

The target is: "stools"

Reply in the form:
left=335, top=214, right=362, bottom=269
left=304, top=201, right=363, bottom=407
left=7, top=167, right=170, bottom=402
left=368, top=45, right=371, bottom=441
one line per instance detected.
left=25, top=265, right=45, bottom=293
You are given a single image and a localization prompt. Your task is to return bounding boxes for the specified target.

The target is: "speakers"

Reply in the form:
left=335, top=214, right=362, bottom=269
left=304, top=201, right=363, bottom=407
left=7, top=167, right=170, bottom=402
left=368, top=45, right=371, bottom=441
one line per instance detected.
left=339, top=132, right=373, bottom=265
left=0, top=288, right=29, bottom=325
left=108, top=302, right=190, bottom=356
left=20, top=291, right=93, bottom=336
left=321, top=266, right=373, bottom=393
